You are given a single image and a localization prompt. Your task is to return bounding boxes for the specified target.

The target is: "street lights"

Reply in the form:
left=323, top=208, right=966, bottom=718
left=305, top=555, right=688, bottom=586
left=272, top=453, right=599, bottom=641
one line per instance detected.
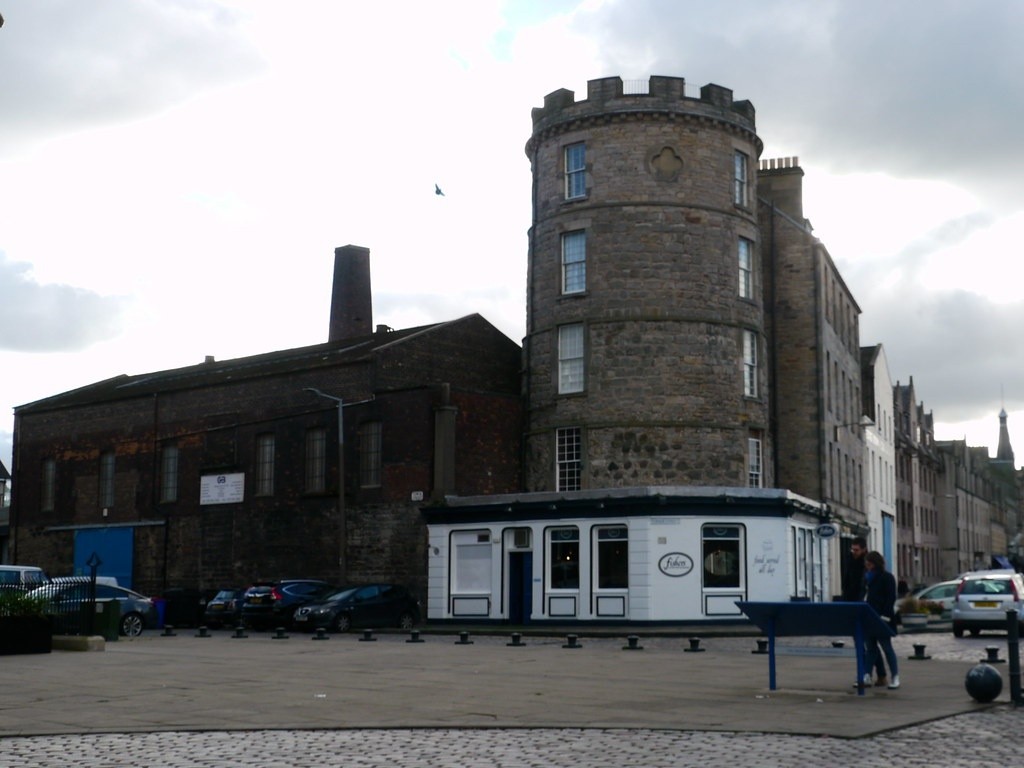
left=303, top=386, right=347, bottom=588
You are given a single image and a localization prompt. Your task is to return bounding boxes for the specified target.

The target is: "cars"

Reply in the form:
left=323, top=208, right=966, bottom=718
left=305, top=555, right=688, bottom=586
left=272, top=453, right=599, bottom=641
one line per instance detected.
left=291, top=583, right=422, bottom=632
left=18, top=581, right=157, bottom=638
left=167, top=588, right=218, bottom=625
left=891, top=569, right=1001, bottom=625
left=204, top=586, right=250, bottom=628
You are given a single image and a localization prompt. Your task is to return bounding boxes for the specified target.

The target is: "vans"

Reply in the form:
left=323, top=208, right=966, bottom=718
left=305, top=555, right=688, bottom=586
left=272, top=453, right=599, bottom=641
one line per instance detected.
left=0, top=564, right=49, bottom=614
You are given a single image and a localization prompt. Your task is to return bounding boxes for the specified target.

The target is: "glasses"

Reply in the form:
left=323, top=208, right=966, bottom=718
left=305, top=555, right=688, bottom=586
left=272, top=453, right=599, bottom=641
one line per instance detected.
left=850, top=548, right=861, bottom=553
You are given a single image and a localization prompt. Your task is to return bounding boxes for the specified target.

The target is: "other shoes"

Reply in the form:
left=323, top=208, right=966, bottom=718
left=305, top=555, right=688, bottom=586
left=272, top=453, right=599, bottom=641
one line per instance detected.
left=874, top=676, right=887, bottom=686
left=888, top=675, right=900, bottom=689
left=853, top=673, right=872, bottom=687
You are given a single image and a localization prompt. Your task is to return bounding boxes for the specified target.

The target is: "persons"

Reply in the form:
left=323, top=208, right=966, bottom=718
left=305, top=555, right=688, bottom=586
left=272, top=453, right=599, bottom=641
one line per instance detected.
left=841, top=537, right=901, bottom=689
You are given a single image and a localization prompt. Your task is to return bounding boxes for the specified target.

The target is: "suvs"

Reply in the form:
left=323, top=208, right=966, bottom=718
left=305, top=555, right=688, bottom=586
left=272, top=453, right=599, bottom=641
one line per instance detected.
left=951, top=573, right=1024, bottom=639
left=239, top=577, right=335, bottom=632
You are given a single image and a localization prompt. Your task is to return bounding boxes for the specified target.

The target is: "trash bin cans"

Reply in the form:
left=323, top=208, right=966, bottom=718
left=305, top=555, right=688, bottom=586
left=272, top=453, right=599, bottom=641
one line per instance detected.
left=102, top=599, right=121, bottom=642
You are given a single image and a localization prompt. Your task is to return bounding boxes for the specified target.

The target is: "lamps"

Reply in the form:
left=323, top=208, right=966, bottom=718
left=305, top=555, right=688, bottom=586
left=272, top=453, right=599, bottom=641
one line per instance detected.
left=838, top=415, right=876, bottom=426
